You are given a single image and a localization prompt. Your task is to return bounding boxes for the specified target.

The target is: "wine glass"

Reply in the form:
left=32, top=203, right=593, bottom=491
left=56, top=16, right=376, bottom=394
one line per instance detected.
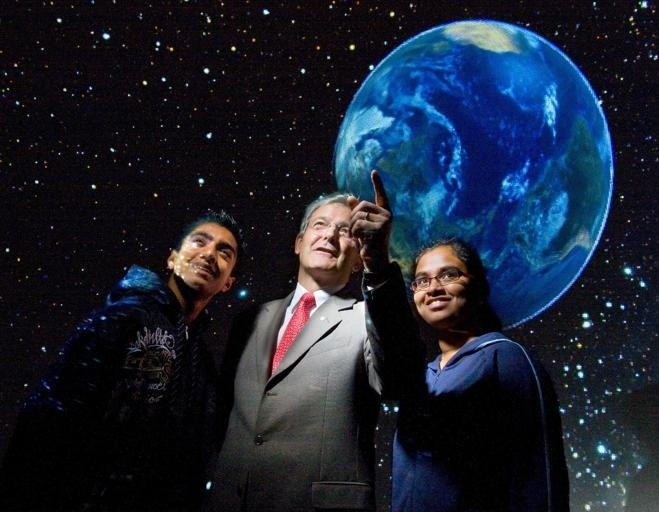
left=364, top=211, right=370, bottom=220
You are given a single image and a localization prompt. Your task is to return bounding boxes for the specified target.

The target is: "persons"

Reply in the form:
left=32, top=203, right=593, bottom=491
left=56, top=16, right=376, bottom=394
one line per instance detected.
left=0, top=212, right=242, bottom=511
left=391, top=235, right=571, bottom=512
left=200, top=170, right=424, bottom=512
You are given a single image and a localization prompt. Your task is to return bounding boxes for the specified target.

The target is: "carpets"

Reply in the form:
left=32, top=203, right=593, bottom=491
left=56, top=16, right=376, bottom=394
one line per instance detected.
left=270, top=293, right=316, bottom=375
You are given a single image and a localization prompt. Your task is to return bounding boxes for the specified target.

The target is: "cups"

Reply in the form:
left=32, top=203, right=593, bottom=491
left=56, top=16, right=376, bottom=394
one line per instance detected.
left=410, top=267, right=481, bottom=292
